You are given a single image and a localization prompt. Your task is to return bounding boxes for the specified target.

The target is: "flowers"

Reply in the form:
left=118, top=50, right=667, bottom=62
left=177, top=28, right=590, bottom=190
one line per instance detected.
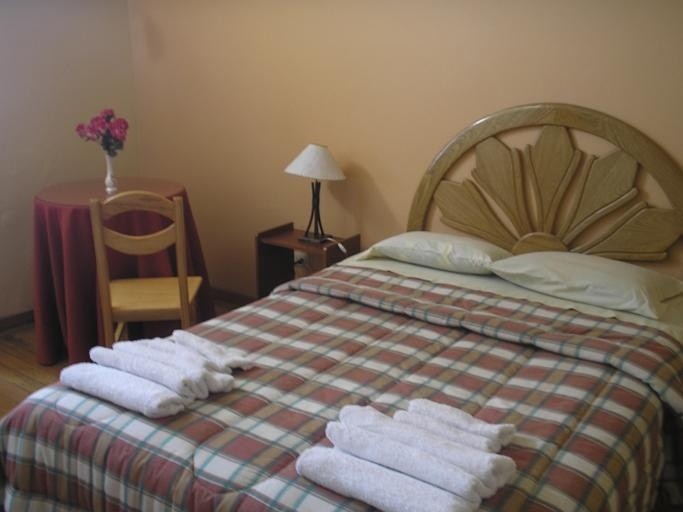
left=79, top=104, right=131, bottom=156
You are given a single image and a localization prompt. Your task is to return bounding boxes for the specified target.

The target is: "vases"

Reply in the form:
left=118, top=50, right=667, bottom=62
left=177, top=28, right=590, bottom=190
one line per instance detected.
left=103, top=153, right=119, bottom=194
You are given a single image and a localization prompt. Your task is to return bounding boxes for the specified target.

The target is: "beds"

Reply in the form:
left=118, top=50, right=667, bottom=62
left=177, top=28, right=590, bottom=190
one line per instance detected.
left=2, top=100, right=683, bottom=512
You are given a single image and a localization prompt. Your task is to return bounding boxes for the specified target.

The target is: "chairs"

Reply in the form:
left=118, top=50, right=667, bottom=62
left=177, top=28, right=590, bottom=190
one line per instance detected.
left=86, top=188, right=203, bottom=347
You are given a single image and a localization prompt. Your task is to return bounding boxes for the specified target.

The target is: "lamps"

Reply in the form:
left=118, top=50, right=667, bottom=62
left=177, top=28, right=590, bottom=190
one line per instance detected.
left=286, top=139, right=344, bottom=243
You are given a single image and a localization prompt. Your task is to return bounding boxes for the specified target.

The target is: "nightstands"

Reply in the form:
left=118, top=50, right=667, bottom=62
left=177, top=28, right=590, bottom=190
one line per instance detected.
left=255, top=220, right=359, bottom=307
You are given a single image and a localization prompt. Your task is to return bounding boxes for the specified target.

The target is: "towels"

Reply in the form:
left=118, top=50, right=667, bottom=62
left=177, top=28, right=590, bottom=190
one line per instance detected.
left=63, top=326, right=255, bottom=419
left=293, top=397, right=523, bottom=512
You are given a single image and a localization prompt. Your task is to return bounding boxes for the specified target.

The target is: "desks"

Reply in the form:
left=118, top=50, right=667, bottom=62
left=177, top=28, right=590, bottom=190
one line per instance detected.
left=30, top=176, right=215, bottom=367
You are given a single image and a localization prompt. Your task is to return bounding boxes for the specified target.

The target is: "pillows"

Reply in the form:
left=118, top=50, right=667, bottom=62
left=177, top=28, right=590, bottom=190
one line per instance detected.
left=360, top=225, right=514, bottom=279
left=487, top=246, right=680, bottom=320
